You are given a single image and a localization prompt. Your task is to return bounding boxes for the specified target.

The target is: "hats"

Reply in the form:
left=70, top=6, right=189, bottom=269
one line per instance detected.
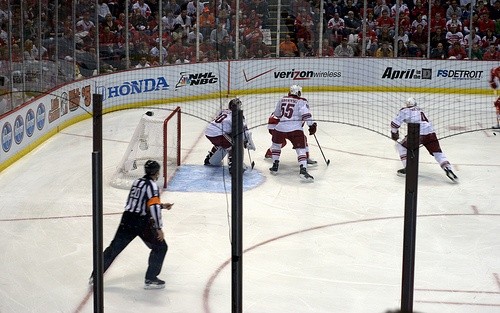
left=341, top=37, right=349, bottom=42
left=64, top=56, right=73, bottom=61
left=155, top=38, right=159, bottom=43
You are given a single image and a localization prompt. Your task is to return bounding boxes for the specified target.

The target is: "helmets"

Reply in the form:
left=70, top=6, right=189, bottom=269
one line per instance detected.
left=289, top=85, right=303, bottom=96
left=406, top=98, right=417, bottom=107
left=144, top=160, right=160, bottom=175
left=228, top=98, right=243, bottom=110
left=450, top=24, right=458, bottom=28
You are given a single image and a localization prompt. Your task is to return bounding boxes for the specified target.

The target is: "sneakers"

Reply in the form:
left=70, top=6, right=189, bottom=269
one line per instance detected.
left=444, top=166, right=459, bottom=183
left=87, top=272, right=94, bottom=289
left=396, top=166, right=406, bottom=177
left=492, top=124, right=500, bottom=132
left=298, top=164, right=314, bottom=183
left=268, top=160, right=280, bottom=175
left=306, top=158, right=318, bottom=168
left=144, top=277, right=166, bottom=289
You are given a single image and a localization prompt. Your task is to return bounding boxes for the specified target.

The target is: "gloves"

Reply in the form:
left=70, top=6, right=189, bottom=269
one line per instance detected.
left=489, top=80, right=498, bottom=90
left=391, top=130, right=400, bottom=141
left=494, top=98, right=500, bottom=107
left=307, top=122, right=317, bottom=136
left=243, top=141, right=249, bottom=149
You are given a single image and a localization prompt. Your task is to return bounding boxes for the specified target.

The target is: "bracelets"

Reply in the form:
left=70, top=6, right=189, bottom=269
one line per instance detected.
left=160, top=204, right=163, bottom=209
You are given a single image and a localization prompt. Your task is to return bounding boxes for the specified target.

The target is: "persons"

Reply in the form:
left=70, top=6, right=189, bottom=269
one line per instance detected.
left=90, top=159, right=174, bottom=289
left=0, top=0, right=274, bottom=118
left=267, top=84, right=317, bottom=182
left=279, top=0, right=500, bottom=61
left=205, top=97, right=256, bottom=175
left=490, top=66, right=500, bottom=129
left=391, top=97, right=459, bottom=183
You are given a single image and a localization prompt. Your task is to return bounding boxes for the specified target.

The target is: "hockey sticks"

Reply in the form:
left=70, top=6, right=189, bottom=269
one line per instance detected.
left=314, top=133, right=330, bottom=166
left=248, top=149, right=255, bottom=169
